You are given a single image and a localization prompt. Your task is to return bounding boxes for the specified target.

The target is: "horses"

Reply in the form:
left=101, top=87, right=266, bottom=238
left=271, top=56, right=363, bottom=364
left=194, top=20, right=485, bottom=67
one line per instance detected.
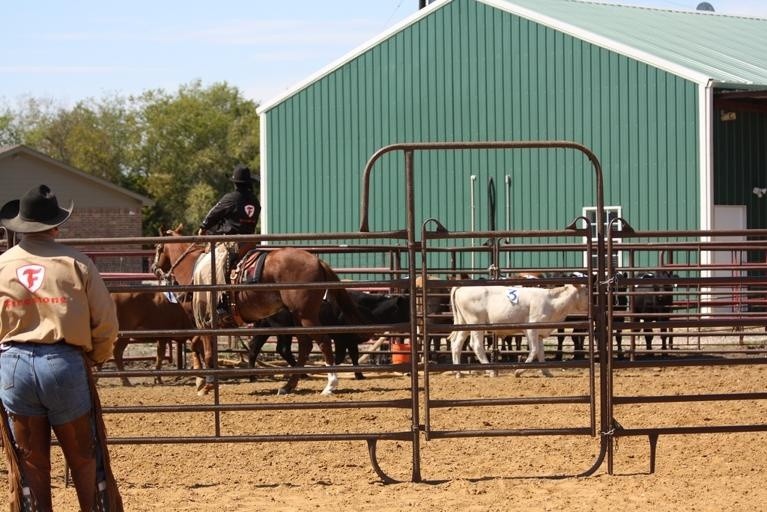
left=150, top=222, right=357, bottom=398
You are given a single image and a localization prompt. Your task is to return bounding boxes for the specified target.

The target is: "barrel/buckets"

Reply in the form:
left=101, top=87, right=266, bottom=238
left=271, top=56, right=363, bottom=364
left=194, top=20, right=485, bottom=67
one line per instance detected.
left=391, top=343, right=422, bottom=365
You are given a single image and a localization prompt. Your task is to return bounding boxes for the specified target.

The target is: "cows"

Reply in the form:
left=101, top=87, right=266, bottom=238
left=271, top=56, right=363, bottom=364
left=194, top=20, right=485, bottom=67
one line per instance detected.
left=410, top=271, right=680, bottom=379
left=92, top=291, right=204, bottom=388
left=247, top=289, right=411, bottom=383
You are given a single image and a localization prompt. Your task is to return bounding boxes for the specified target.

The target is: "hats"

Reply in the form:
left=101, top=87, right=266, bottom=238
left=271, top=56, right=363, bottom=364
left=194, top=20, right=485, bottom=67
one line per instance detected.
left=1, top=184, right=75, bottom=234
left=229, top=167, right=253, bottom=184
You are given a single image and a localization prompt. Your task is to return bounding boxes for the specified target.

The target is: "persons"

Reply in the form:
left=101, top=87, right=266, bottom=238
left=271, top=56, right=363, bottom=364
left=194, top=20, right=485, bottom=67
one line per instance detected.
left=195, top=166, right=261, bottom=316
left=0, top=184, right=122, bottom=512
